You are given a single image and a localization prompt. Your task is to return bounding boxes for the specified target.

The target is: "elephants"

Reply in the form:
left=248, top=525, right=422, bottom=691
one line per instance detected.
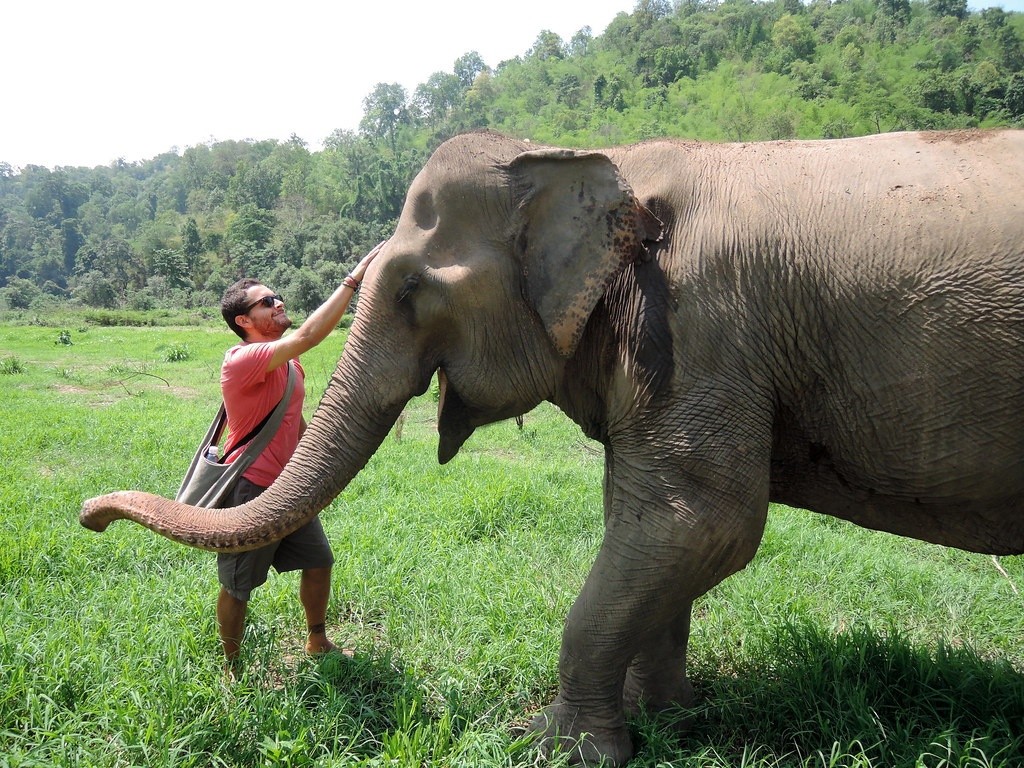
left=78, top=125, right=1024, bottom=768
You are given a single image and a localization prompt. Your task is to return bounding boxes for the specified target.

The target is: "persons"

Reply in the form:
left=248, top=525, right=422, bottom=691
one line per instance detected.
left=217, top=238, right=389, bottom=677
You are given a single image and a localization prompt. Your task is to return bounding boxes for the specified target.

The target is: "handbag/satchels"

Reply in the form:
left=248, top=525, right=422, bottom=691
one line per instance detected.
left=174, top=418, right=254, bottom=510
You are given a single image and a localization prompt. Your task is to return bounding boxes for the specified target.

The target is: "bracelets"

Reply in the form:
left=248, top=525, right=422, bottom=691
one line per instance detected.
left=342, top=275, right=361, bottom=292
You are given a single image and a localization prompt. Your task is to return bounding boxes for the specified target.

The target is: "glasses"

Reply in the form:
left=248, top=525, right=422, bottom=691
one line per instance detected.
left=244, top=293, right=285, bottom=312
